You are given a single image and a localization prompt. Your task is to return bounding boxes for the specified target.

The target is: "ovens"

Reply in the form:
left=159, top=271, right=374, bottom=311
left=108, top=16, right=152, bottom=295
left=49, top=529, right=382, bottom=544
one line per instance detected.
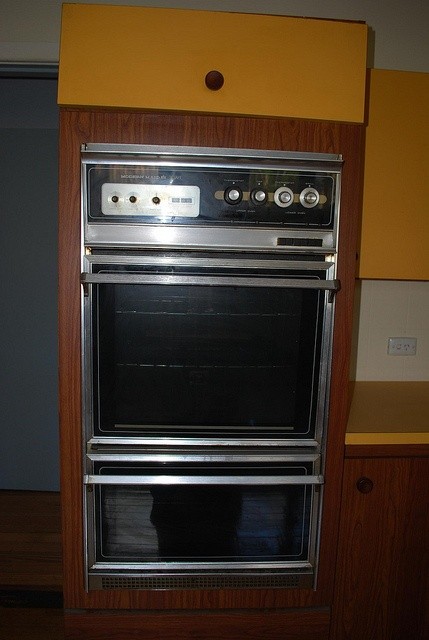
left=82, top=254, right=342, bottom=597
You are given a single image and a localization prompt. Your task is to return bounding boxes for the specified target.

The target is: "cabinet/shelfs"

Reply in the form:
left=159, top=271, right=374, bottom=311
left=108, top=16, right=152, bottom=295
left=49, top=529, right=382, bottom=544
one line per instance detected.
left=333, top=429, right=426, bottom=639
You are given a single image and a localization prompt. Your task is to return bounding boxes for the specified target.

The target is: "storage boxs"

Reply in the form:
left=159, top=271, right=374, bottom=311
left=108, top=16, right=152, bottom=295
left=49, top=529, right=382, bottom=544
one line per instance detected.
left=356, top=69, right=429, bottom=279
left=57, top=2, right=368, bottom=125
